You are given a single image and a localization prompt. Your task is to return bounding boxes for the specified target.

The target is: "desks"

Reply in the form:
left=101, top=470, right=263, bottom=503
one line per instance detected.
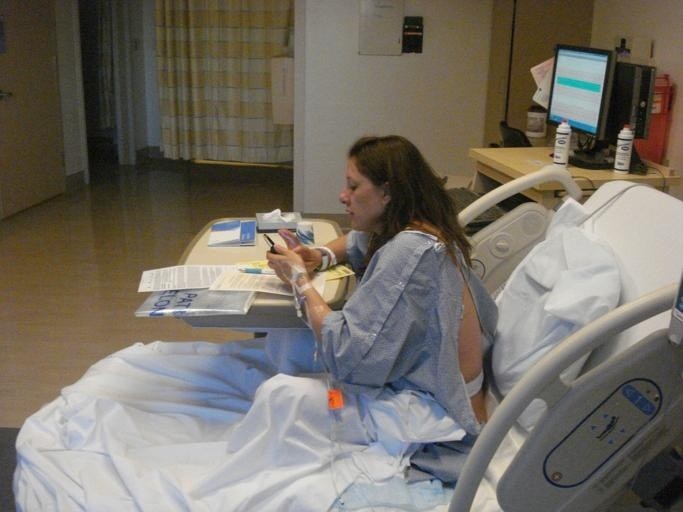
left=177, top=217, right=358, bottom=329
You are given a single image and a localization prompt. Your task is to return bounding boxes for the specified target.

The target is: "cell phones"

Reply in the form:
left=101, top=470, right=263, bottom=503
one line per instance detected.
left=263, top=234, right=275, bottom=254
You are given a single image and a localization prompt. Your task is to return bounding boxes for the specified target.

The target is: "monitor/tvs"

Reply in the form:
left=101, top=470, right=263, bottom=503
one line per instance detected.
left=605, top=62, right=657, bottom=175
left=545, top=44, right=617, bottom=169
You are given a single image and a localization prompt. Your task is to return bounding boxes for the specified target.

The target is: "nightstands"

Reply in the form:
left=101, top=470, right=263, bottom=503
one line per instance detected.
left=465, top=144, right=683, bottom=216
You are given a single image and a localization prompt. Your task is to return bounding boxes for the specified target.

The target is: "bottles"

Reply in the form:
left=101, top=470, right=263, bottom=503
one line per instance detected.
left=552, top=119, right=573, bottom=170
left=612, top=125, right=634, bottom=174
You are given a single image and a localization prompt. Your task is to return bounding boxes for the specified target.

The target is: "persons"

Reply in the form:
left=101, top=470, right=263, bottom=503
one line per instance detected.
left=243, top=136, right=496, bottom=446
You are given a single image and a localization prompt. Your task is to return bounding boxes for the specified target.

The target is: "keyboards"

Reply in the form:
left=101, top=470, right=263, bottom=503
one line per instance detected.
left=445, top=188, right=505, bottom=236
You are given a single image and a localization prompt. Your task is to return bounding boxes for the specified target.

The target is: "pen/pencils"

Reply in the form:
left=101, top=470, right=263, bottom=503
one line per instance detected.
left=238, top=267, right=275, bottom=275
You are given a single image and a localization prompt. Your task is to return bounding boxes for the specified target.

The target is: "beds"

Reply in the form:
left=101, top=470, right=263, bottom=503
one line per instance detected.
left=17, top=163, right=683, bottom=512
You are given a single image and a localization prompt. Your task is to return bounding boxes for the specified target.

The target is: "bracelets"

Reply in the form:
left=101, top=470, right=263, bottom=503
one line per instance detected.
left=312, top=248, right=330, bottom=273
